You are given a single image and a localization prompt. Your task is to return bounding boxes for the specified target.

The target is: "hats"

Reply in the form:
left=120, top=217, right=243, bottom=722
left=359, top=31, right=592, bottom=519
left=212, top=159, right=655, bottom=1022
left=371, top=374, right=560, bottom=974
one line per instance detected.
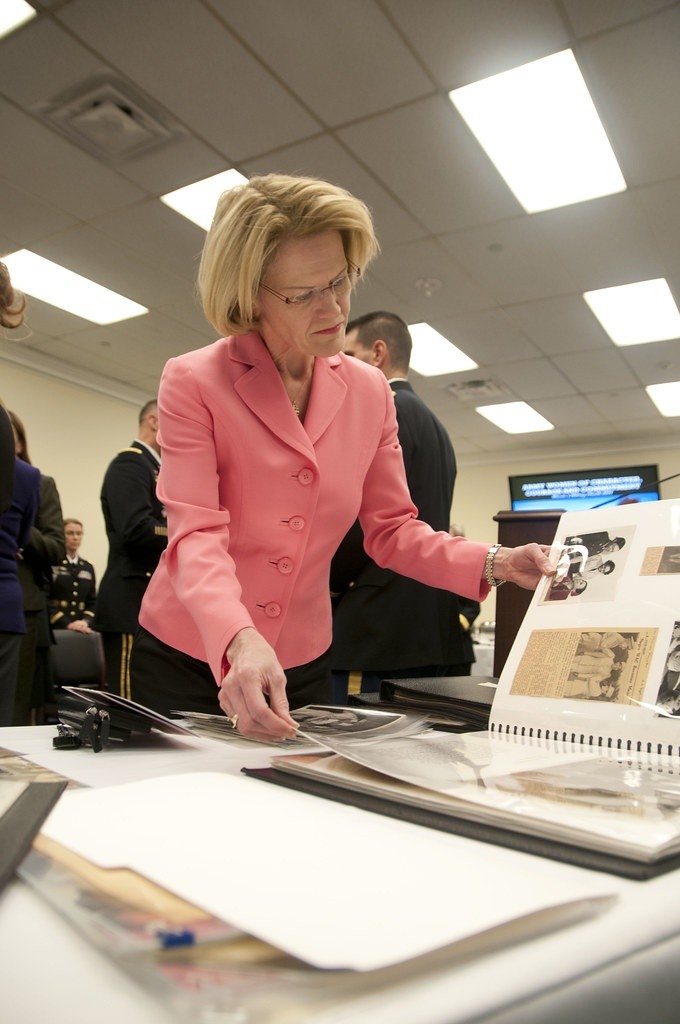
left=619, top=661, right=625, bottom=673
left=627, top=636, right=634, bottom=650
left=605, top=684, right=615, bottom=698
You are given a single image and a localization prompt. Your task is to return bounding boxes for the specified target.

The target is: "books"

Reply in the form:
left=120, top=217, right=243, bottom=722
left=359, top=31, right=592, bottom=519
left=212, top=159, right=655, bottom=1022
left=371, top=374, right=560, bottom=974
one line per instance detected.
left=237, top=498, right=680, bottom=881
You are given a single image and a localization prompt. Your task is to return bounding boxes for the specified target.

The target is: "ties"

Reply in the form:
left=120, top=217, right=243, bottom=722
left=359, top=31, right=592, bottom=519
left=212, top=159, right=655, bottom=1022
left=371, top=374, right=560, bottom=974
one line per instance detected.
left=71, top=563, right=77, bottom=579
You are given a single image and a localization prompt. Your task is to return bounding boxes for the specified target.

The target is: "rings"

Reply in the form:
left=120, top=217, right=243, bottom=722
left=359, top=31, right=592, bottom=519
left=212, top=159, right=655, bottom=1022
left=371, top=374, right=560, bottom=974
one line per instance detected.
left=227, top=715, right=238, bottom=728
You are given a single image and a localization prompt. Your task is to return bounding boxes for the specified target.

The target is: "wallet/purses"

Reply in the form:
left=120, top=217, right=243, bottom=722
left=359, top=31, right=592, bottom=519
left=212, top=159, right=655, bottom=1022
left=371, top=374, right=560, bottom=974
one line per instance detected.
left=57, top=696, right=153, bottom=753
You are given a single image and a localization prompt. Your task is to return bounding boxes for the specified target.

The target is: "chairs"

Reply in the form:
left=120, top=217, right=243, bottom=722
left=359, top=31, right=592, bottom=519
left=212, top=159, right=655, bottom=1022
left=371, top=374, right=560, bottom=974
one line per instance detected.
left=45, top=626, right=105, bottom=726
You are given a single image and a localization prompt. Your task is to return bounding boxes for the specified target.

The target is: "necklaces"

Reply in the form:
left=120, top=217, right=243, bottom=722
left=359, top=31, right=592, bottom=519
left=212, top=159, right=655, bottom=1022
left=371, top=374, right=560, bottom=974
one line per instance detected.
left=290, top=373, right=312, bottom=417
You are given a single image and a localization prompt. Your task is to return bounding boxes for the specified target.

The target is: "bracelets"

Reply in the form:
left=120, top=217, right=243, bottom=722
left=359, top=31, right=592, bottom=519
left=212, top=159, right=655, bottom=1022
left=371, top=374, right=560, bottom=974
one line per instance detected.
left=486, top=544, right=507, bottom=587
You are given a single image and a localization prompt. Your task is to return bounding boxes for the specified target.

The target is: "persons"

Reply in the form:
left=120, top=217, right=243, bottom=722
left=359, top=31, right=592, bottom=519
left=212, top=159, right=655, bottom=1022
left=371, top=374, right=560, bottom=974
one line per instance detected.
left=328, top=311, right=480, bottom=706
left=46, top=520, right=106, bottom=690
left=546, top=531, right=625, bottom=601
left=288, top=707, right=366, bottom=729
left=0, top=401, right=68, bottom=728
left=565, top=632, right=635, bottom=700
left=88, top=397, right=167, bottom=706
left=128, top=175, right=555, bottom=741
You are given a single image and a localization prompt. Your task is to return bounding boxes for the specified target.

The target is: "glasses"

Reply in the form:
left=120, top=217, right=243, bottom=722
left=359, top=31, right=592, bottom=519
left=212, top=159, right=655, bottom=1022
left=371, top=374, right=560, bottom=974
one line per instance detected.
left=259, top=256, right=360, bottom=312
